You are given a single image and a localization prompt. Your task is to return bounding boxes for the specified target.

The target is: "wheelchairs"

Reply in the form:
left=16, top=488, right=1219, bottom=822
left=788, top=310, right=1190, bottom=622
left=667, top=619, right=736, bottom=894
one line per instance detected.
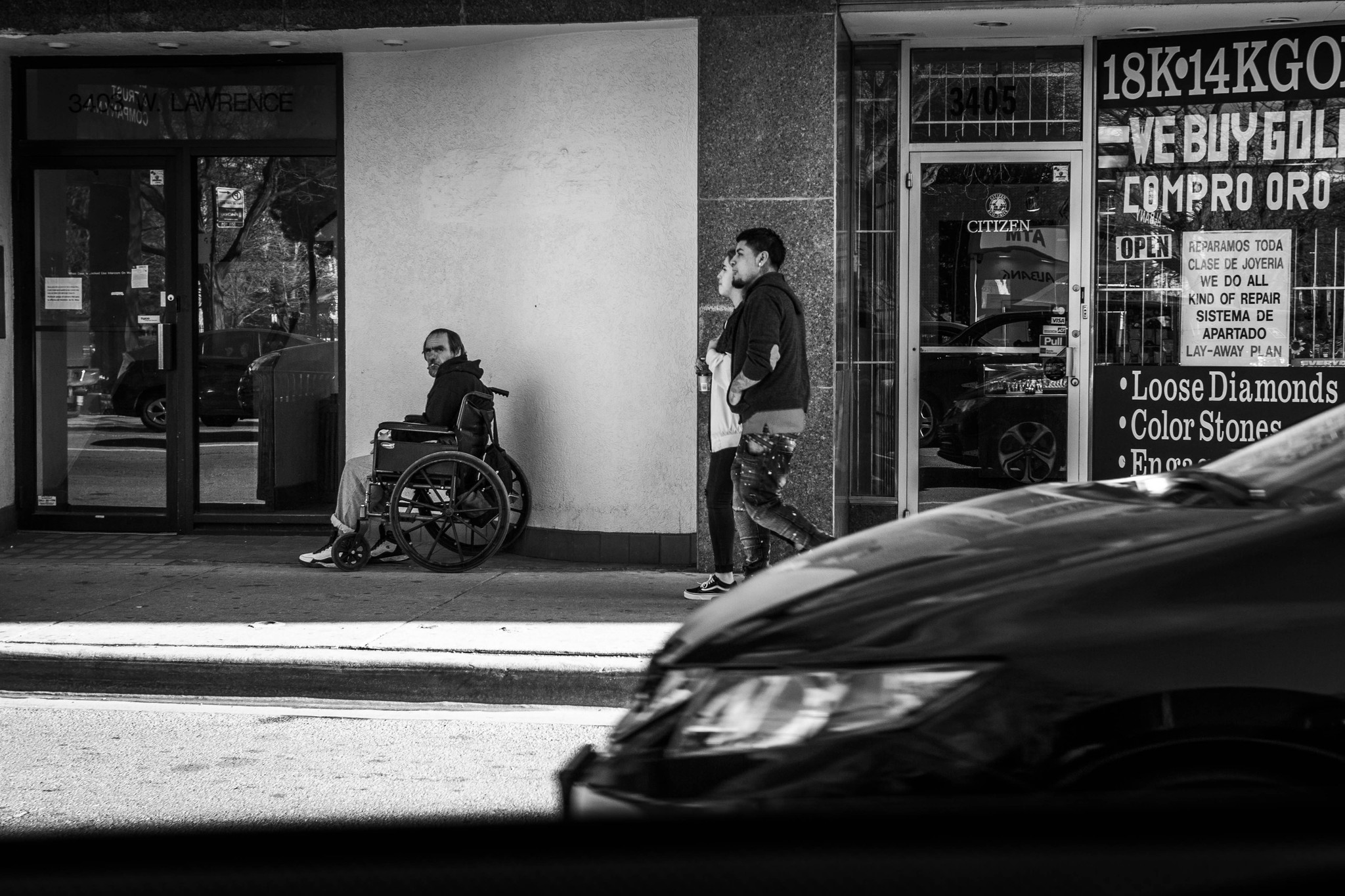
left=331, top=387, right=532, bottom=575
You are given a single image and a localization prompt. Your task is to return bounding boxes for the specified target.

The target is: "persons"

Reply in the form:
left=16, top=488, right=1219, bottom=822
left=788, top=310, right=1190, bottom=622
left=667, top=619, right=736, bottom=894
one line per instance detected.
left=714, top=228, right=840, bottom=586
left=683, top=250, right=744, bottom=600
left=298, top=328, right=496, bottom=568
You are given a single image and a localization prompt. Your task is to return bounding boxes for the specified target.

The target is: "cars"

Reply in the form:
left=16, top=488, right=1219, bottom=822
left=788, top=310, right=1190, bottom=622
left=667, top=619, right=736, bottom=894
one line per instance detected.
left=110, top=329, right=330, bottom=432
left=913, top=300, right=1178, bottom=487
left=558, top=403, right=1345, bottom=820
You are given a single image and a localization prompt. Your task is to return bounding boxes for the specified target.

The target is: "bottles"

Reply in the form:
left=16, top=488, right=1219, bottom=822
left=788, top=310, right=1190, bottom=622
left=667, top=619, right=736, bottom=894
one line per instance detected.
left=697, top=356, right=712, bottom=398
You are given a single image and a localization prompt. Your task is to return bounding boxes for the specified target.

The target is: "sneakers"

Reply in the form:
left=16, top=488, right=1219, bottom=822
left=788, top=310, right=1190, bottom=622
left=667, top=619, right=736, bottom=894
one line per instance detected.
left=683, top=573, right=737, bottom=599
left=298, top=531, right=357, bottom=568
left=368, top=524, right=412, bottom=562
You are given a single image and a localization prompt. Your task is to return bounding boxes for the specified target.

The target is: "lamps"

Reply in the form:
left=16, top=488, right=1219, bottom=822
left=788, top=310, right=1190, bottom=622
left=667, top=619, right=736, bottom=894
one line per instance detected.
left=1025, top=191, right=1040, bottom=212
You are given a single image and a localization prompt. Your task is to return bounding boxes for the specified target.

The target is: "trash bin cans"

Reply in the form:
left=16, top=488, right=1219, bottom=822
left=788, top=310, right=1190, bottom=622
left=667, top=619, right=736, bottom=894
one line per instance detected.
left=247, top=370, right=341, bottom=512
